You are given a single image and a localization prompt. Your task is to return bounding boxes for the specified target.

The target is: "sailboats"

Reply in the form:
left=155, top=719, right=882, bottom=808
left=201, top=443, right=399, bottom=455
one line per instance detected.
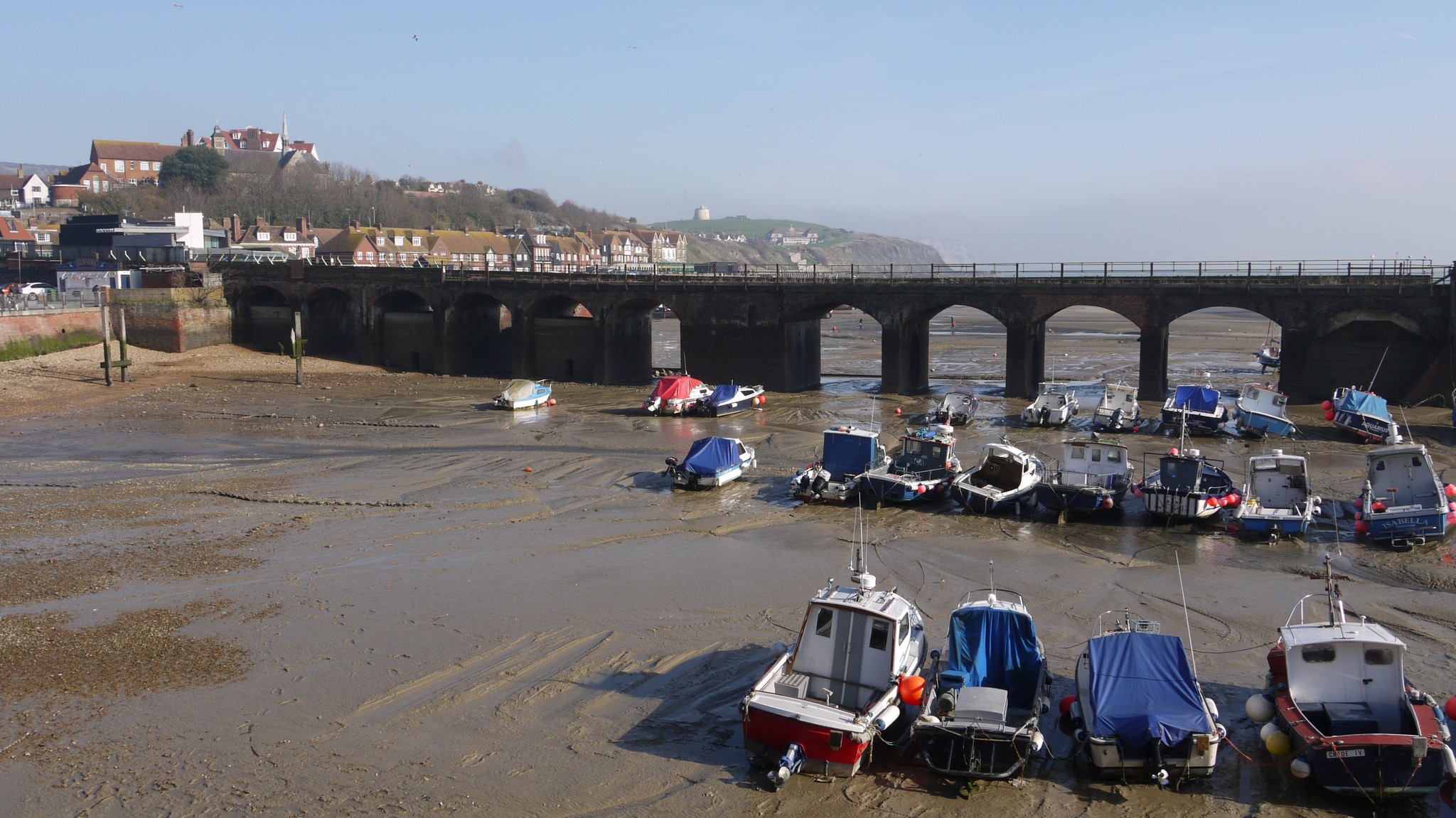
left=1258, top=318, right=1282, bottom=368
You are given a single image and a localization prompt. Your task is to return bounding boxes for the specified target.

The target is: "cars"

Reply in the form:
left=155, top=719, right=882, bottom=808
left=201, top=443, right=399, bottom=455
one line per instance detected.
left=0, top=282, right=24, bottom=297
left=19, top=282, right=58, bottom=300
left=638, top=270, right=654, bottom=275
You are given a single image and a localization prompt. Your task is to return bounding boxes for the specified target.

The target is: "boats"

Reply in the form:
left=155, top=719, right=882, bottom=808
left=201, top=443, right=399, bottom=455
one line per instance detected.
left=1320, top=346, right=1400, bottom=443
left=1238, top=449, right=1321, bottom=543
left=492, top=379, right=554, bottom=411
left=740, top=488, right=931, bottom=794
left=660, top=436, right=757, bottom=490
left=1033, top=431, right=1135, bottom=515
left=898, top=558, right=1053, bottom=802
left=1231, top=381, right=1296, bottom=441
left=689, top=380, right=766, bottom=419
left=640, top=352, right=714, bottom=416
left=1354, top=406, right=1456, bottom=551
left=1090, top=372, right=1142, bottom=434
left=1059, top=548, right=1228, bottom=787
left=1245, top=549, right=1456, bottom=807
left=1130, top=401, right=1242, bottom=523
left=1158, top=371, right=1229, bottom=434
left=854, top=402, right=962, bottom=506
left=1020, top=381, right=1080, bottom=427
left=935, top=388, right=979, bottom=427
left=789, top=396, right=900, bottom=505
left=952, top=434, right=1062, bottom=513
